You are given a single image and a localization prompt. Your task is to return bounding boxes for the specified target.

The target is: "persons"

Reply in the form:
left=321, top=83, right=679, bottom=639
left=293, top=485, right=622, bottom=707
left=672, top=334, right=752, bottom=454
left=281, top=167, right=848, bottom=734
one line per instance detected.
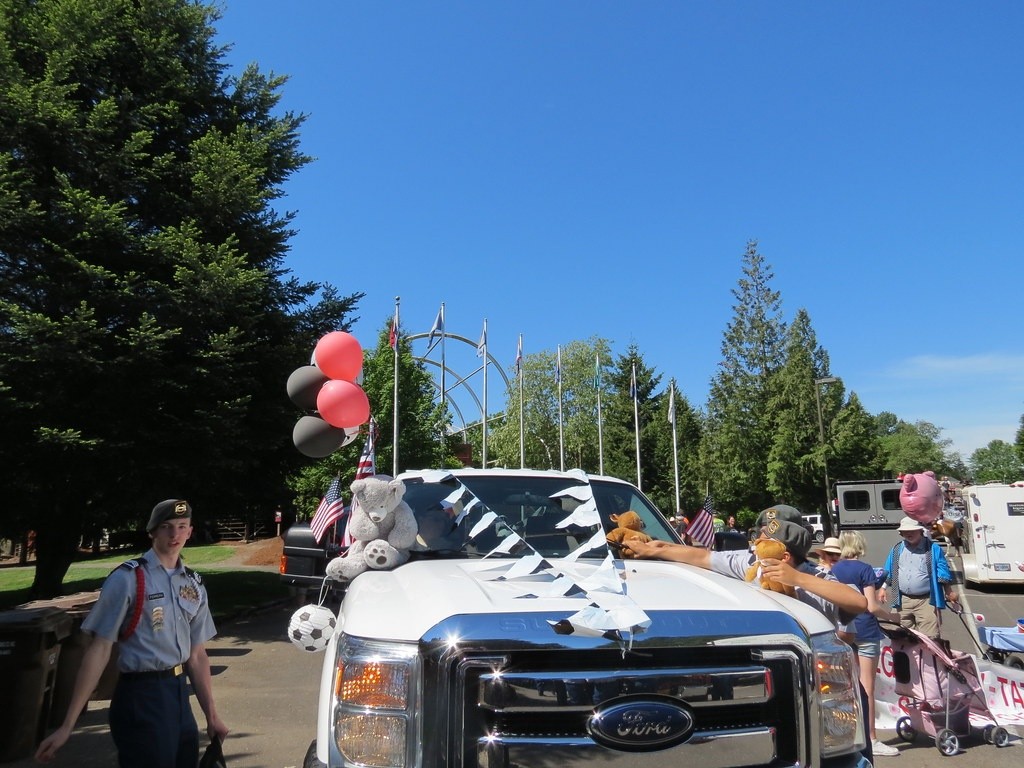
left=620, top=503, right=899, bottom=768
left=875, top=517, right=959, bottom=644
left=36, top=499, right=230, bottom=768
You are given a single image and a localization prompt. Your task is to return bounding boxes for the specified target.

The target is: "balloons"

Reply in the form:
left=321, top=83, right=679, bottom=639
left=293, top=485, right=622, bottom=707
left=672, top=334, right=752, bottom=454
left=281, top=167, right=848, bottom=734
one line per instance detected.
left=285, top=329, right=371, bottom=458
left=899, top=470, right=944, bottom=524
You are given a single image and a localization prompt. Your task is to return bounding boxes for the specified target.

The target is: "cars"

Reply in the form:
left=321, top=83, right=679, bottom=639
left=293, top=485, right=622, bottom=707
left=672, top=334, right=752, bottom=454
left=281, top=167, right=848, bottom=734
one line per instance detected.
left=747, top=525, right=758, bottom=543
left=953, top=481, right=1024, bottom=590
left=730, top=528, right=746, bottom=534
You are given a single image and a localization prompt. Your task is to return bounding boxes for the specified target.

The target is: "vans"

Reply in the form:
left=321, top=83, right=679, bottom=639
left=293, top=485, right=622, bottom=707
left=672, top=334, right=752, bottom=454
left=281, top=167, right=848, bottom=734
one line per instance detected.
left=801, top=514, right=839, bottom=544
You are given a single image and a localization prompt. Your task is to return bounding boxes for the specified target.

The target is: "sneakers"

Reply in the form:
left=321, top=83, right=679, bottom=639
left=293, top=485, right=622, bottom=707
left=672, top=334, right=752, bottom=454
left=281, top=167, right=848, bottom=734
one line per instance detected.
left=871, top=740, right=899, bottom=755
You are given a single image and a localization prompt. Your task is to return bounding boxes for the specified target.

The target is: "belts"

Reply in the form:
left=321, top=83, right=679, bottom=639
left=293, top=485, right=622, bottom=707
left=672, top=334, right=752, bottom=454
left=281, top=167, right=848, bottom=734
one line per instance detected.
left=122, top=664, right=187, bottom=679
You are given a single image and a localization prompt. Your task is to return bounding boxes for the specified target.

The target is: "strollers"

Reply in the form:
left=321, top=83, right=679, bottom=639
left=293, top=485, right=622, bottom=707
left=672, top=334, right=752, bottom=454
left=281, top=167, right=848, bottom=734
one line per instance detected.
left=875, top=618, right=1009, bottom=755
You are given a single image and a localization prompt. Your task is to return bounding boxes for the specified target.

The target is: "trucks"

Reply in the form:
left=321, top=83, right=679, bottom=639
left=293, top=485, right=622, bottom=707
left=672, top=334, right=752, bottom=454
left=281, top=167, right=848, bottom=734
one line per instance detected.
left=277, top=467, right=878, bottom=768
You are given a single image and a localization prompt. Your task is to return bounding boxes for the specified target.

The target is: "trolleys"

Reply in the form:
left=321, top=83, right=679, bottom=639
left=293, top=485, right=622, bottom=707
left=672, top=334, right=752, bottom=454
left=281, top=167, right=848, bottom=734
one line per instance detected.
left=943, top=600, right=1024, bottom=671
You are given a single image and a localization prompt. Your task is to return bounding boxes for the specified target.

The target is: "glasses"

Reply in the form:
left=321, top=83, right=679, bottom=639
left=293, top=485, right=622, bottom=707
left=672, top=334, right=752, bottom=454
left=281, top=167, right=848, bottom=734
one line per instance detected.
left=825, top=552, right=841, bottom=556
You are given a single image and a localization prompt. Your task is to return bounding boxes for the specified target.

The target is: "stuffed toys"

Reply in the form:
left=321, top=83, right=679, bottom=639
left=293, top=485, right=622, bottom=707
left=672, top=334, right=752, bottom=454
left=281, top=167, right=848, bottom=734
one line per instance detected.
left=325, top=477, right=417, bottom=583
left=606, top=511, right=653, bottom=561
left=745, top=539, right=792, bottom=594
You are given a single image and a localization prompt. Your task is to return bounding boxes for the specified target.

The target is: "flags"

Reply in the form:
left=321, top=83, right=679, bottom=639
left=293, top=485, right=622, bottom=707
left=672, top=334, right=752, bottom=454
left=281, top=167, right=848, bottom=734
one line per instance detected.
left=425, top=308, right=443, bottom=348
left=339, top=432, right=374, bottom=556
left=475, top=328, right=487, bottom=358
left=686, top=498, right=716, bottom=549
left=310, top=479, right=344, bottom=545
left=390, top=314, right=401, bottom=355
left=593, top=363, right=599, bottom=390
left=514, top=343, right=522, bottom=376
left=630, top=370, right=642, bottom=405
left=667, top=394, right=673, bottom=423
left=555, top=360, right=560, bottom=384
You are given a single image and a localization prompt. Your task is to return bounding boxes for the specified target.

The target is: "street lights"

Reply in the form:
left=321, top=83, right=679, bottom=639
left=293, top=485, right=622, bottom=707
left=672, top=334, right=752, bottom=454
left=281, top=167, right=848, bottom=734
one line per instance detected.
left=816, top=379, right=839, bottom=538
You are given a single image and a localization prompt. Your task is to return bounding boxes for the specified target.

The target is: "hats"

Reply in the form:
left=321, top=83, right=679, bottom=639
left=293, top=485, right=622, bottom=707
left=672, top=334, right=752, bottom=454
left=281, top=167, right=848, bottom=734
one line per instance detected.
left=755, top=505, right=814, bottom=553
left=146, top=499, right=192, bottom=531
left=896, top=517, right=923, bottom=531
left=815, top=537, right=841, bottom=556
